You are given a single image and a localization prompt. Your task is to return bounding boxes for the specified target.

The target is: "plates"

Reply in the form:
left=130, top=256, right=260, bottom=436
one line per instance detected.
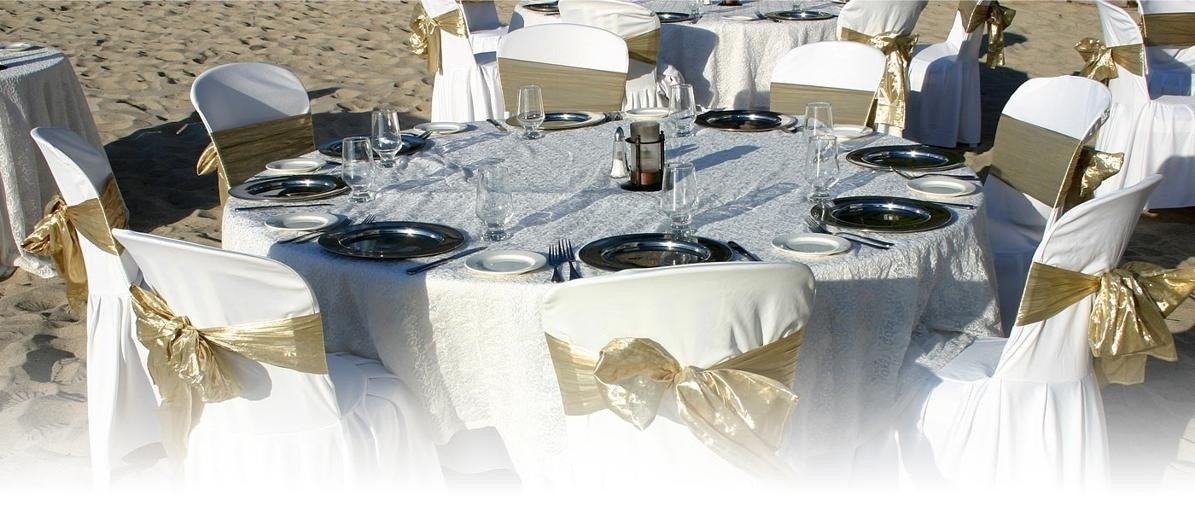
left=625, top=107, right=675, bottom=119
left=264, top=212, right=340, bottom=231
left=904, top=174, right=978, bottom=196
left=0, top=42, right=36, bottom=52
left=844, top=144, right=966, bottom=172
left=504, top=108, right=606, bottom=130
left=319, top=220, right=465, bottom=261
left=771, top=231, right=851, bottom=257
left=522, top=2, right=560, bottom=12
left=464, top=248, right=547, bottom=276
left=765, top=9, right=834, bottom=20
left=225, top=174, right=349, bottom=202
left=318, top=132, right=426, bottom=160
left=822, top=123, right=874, bottom=139
left=720, top=15, right=754, bottom=21
left=655, top=12, right=702, bottom=23
left=578, top=232, right=735, bottom=273
left=692, top=108, right=799, bottom=133
left=413, top=122, right=469, bottom=134
left=808, top=194, right=954, bottom=234
left=264, top=157, right=328, bottom=173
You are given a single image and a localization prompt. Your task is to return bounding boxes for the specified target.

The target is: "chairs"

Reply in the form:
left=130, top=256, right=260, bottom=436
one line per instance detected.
left=417, top=1, right=1195, bottom=330
left=880, top=171, right=1162, bottom=480
left=29, top=124, right=179, bottom=483
left=110, top=225, right=423, bottom=483
left=188, top=61, right=314, bottom=202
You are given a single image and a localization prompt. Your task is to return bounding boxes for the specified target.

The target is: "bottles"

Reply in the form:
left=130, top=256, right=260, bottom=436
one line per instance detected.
left=608, top=126, right=630, bottom=178
left=703, top=0, right=712, bottom=6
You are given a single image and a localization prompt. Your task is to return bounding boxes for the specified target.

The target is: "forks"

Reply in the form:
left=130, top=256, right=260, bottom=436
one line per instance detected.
left=754, top=10, right=782, bottom=23
left=275, top=217, right=353, bottom=245
left=293, top=214, right=376, bottom=245
left=890, top=164, right=976, bottom=181
left=802, top=215, right=895, bottom=251
left=547, top=242, right=562, bottom=283
left=557, top=239, right=582, bottom=282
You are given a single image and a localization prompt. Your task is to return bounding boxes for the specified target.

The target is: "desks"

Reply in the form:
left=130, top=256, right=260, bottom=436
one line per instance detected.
left=222, top=105, right=997, bottom=481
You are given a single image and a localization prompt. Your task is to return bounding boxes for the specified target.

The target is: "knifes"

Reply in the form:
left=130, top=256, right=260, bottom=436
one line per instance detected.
left=727, top=239, right=761, bottom=261
left=831, top=2, right=846, bottom=5
left=234, top=204, right=332, bottom=211
left=406, top=246, right=486, bottom=276
left=487, top=119, right=507, bottom=133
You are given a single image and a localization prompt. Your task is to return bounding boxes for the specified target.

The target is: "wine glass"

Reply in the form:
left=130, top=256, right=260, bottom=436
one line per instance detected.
left=668, top=82, right=697, bottom=139
left=340, top=136, right=375, bottom=205
left=370, top=110, right=403, bottom=171
left=803, top=102, right=834, bottom=162
left=657, top=234, right=704, bottom=266
left=791, top=0, right=803, bottom=11
left=803, top=134, right=841, bottom=205
left=474, top=164, right=516, bottom=241
left=660, top=159, right=697, bottom=234
left=686, top=0, right=701, bottom=27
left=515, top=85, right=545, bottom=141
